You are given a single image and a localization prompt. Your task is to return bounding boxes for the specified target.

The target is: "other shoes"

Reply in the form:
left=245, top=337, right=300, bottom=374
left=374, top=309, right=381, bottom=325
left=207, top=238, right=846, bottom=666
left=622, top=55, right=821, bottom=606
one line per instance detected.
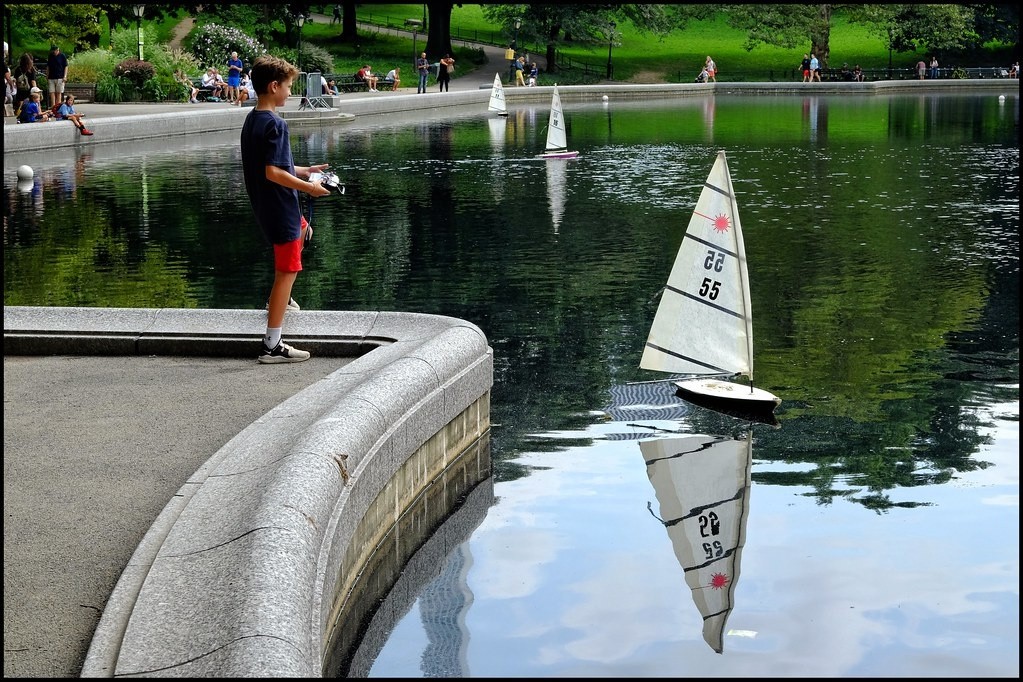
left=369, top=88, right=379, bottom=92
left=191, top=98, right=200, bottom=104
left=81, top=129, right=93, bottom=135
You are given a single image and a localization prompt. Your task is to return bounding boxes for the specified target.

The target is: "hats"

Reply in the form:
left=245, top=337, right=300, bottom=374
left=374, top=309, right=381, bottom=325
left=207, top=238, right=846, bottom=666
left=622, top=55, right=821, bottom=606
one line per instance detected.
left=50, top=43, right=58, bottom=50
left=30, top=86, right=43, bottom=93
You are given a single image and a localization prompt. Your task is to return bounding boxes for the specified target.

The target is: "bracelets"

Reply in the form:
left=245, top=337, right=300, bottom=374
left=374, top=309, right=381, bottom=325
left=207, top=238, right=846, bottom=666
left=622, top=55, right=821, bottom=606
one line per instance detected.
left=308, top=182, right=316, bottom=195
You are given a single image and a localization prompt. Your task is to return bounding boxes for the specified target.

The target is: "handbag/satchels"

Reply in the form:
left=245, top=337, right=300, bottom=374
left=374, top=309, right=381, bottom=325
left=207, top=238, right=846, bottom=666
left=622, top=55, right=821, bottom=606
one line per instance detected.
left=20, top=113, right=30, bottom=123
left=713, top=62, right=716, bottom=68
left=4, top=103, right=15, bottom=117
left=206, top=95, right=221, bottom=102
left=353, top=73, right=363, bottom=82
left=446, top=64, right=455, bottom=73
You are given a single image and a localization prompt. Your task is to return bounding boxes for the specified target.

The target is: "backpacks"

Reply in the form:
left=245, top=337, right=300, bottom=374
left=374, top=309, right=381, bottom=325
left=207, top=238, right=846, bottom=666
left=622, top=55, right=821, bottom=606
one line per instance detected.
left=16, top=68, right=31, bottom=92
left=51, top=102, right=68, bottom=117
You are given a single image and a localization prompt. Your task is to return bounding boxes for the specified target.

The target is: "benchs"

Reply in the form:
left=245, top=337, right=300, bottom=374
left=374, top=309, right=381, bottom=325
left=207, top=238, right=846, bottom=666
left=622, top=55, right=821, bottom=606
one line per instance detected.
left=187, top=78, right=243, bottom=102
left=829, top=69, right=867, bottom=81
left=323, top=73, right=394, bottom=94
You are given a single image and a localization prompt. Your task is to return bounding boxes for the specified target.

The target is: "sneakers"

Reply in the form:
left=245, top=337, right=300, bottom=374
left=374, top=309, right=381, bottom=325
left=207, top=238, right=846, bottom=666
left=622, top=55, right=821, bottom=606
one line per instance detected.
left=258, top=337, right=311, bottom=364
left=265, top=295, right=301, bottom=311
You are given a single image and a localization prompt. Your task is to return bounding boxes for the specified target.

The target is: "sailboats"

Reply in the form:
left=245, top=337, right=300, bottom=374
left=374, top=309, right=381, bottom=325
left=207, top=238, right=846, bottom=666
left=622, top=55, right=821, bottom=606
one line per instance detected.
left=534, top=83, right=580, bottom=159
left=636, top=147, right=785, bottom=429
left=488, top=72, right=509, bottom=116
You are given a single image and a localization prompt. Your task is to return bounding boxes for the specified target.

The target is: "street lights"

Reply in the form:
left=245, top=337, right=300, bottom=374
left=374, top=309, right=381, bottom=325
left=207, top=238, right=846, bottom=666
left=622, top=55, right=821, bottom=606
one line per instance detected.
left=508, top=17, right=522, bottom=82
left=132, top=2, right=146, bottom=89
left=606, top=19, right=617, bottom=81
left=888, top=29, right=895, bottom=79
left=294, top=11, right=306, bottom=93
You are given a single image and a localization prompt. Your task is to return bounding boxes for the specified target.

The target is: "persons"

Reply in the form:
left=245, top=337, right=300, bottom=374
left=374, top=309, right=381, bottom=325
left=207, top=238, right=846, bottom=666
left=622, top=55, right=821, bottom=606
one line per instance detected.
left=530, top=62, right=539, bottom=86
left=698, top=56, right=716, bottom=83
left=1009, top=63, right=1017, bottom=79
left=508, top=49, right=529, bottom=86
left=798, top=52, right=811, bottom=82
left=46, top=45, right=69, bottom=118
left=57, top=94, right=93, bottom=135
left=231, top=69, right=257, bottom=105
left=809, top=53, right=821, bottom=82
left=853, top=63, right=866, bottom=82
left=930, top=56, right=938, bottom=79
left=417, top=51, right=429, bottom=94
left=357, top=65, right=379, bottom=92
left=1014, top=61, right=1019, bottom=78
left=227, top=51, right=243, bottom=106
left=241, top=54, right=331, bottom=364
left=202, top=67, right=230, bottom=102
left=841, top=62, right=853, bottom=82
left=437, top=54, right=455, bottom=92
left=174, top=70, right=199, bottom=103
left=915, top=59, right=926, bottom=80
left=4, top=42, right=53, bottom=124
left=299, top=69, right=332, bottom=106
left=327, top=79, right=339, bottom=96
left=385, top=67, right=401, bottom=91
